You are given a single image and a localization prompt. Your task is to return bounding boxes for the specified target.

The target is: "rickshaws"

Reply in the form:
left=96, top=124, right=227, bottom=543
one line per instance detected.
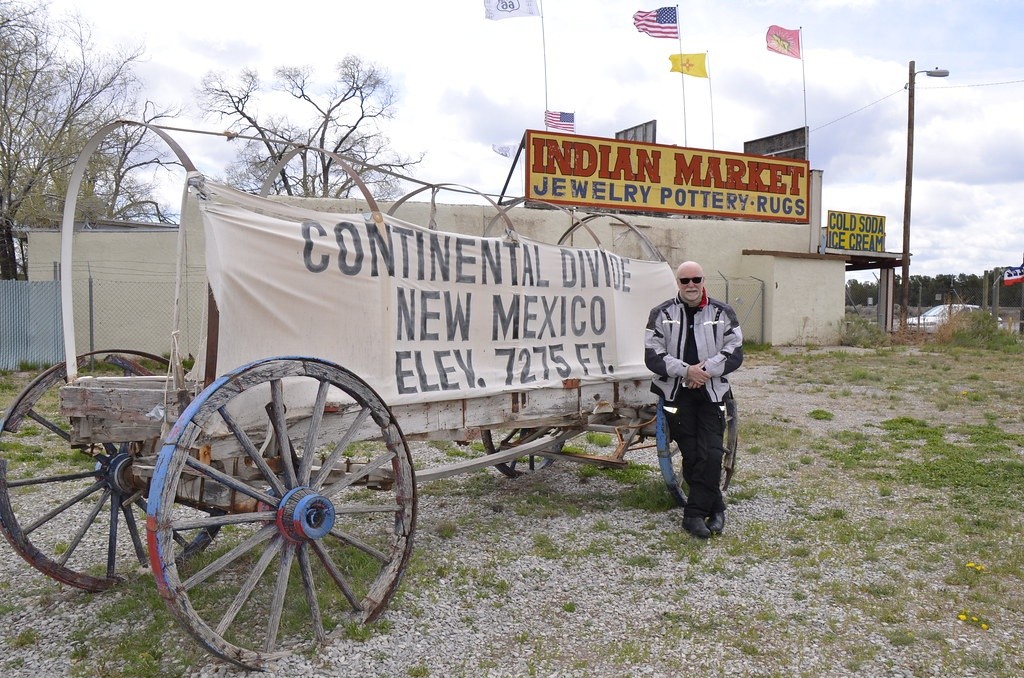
left=0, top=120, right=739, bottom=672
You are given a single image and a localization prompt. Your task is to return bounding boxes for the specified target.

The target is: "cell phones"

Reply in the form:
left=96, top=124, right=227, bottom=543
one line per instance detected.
left=682, top=378, right=689, bottom=387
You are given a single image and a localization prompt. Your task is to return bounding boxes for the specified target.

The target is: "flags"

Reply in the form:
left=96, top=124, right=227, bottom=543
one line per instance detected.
left=669, top=53, right=708, bottom=78
left=544, top=110, right=574, bottom=132
left=484, top=0, right=540, bottom=20
left=492, top=144, right=518, bottom=160
left=633, top=7, right=679, bottom=39
left=766, top=25, right=801, bottom=59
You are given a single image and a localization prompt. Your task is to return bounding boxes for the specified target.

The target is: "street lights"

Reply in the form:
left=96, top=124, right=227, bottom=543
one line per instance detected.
left=899, top=61, right=949, bottom=335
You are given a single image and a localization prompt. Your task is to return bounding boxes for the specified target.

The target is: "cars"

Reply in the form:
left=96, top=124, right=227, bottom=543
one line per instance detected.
left=906, top=304, right=1003, bottom=334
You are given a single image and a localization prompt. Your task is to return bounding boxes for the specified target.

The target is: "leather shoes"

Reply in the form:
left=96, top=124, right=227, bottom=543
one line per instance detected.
left=706, top=512, right=725, bottom=533
left=682, top=518, right=711, bottom=538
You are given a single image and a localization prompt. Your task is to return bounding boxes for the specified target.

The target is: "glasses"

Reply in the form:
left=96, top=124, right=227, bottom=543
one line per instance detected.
left=678, top=277, right=702, bottom=284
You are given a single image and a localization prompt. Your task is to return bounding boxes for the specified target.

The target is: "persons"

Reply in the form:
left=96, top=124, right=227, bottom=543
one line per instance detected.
left=644, top=261, right=744, bottom=538
